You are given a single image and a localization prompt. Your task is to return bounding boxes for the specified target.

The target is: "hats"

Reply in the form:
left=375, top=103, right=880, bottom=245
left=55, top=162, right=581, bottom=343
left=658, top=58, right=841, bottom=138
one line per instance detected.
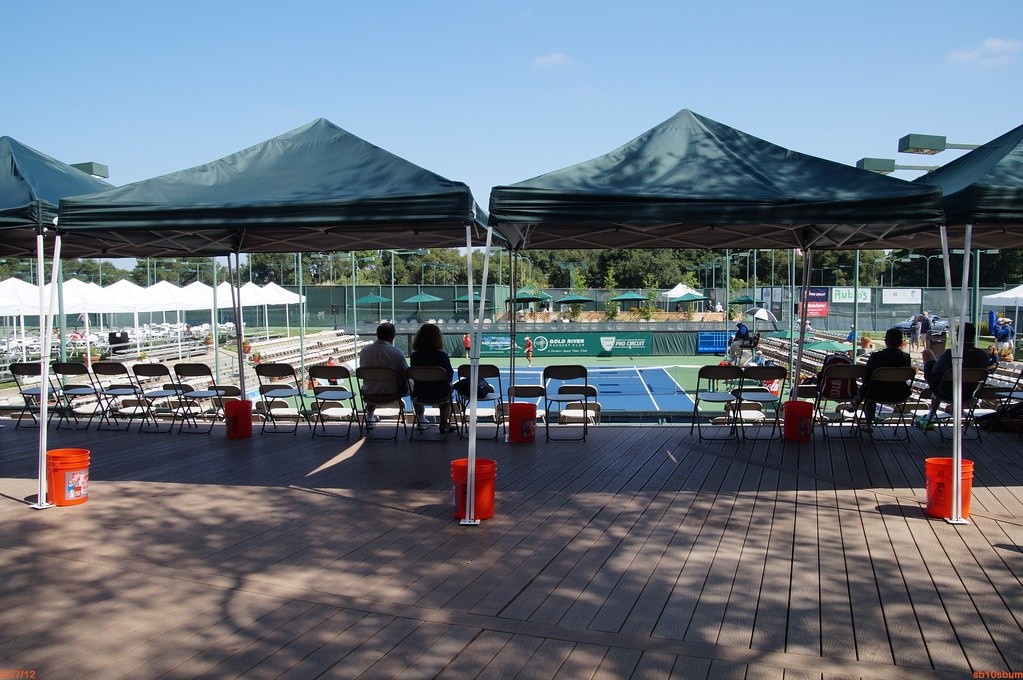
left=735, top=321, right=742, bottom=327
left=797, top=319, right=810, bottom=324
left=998, top=317, right=1013, bottom=323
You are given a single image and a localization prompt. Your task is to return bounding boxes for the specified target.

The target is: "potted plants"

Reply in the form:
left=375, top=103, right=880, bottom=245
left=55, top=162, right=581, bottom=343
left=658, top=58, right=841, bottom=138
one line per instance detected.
left=900, top=334, right=908, bottom=350
left=861, top=329, right=871, bottom=348
left=83, top=346, right=100, bottom=368
left=270, top=360, right=279, bottom=382
left=253, top=351, right=261, bottom=363
left=815, top=366, right=822, bottom=377
left=205, top=334, right=213, bottom=345
left=309, top=377, right=317, bottom=390
left=243, top=339, right=252, bottom=353
left=297, top=376, right=309, bottom=390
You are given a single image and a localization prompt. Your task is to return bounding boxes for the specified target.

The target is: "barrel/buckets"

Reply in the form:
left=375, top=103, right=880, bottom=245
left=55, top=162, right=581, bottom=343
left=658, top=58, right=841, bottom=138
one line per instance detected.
left=225, top=400, right=252, bottom=438
left=451, top=459, right=497, bottom=519
left=509, top=403, right=537, bottom=443
left=925, top=458, right=974, bottom=518
left=784, top=401, right=813, bottom=441
left=46, top=448, right=91, bottom=506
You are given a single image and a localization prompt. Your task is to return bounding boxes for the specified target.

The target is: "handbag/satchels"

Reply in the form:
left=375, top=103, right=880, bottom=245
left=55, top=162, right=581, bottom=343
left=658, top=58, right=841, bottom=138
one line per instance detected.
left=453, top=376, right=494, bottom=399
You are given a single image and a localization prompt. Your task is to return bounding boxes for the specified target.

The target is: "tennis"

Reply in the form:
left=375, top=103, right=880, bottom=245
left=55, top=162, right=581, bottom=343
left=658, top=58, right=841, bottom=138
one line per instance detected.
left=690, top=394, right=693, bottom=397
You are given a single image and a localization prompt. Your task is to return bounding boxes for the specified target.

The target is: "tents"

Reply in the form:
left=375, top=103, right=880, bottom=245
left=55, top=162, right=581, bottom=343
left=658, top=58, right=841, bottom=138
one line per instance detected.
left=0, top=108, right=1023, bottom=525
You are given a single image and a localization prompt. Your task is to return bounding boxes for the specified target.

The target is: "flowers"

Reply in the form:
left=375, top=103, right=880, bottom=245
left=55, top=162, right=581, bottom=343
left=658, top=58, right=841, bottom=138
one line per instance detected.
left=999, top=346, right=1013, bottom=357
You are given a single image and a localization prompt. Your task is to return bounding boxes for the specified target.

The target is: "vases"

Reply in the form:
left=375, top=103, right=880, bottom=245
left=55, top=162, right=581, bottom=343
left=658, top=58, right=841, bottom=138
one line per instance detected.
left=1001, top=355, right=1013, bottom=362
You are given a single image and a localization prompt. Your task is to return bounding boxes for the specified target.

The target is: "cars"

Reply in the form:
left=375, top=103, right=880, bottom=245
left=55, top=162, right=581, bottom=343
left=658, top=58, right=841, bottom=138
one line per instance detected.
left=894, top=314, right=950, bottom=335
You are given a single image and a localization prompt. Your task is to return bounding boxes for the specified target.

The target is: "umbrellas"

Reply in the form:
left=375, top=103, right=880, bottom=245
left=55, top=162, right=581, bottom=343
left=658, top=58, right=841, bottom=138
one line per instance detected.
left=670, top=293, right=709, bottom=302
left=728, top=294, right=766, bottom=317
left=744, top=308, right=778, bottom=334
left=400, top=291, right=444, bottom=319
left=554, top=293, right=594, bottom=304
left=452, top=292, right=491, bottom=302
left=609, top=291, right=650, bottom=321
left=351, top=293, right=392, bottom=317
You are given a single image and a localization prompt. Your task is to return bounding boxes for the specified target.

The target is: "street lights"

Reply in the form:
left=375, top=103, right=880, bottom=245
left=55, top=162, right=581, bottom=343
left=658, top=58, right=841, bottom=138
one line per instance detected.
left=874, top=258, right=911, bottom=287
left=908, top=253, right=943, bottom=287
left=11, top=217, right=854, bottom=322
left=950, top=248, right=999, bottom=348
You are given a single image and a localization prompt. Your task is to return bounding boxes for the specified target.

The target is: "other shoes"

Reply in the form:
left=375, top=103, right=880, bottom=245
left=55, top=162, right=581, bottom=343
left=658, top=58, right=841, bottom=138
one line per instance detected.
left=417, top=419, right=430, bottom=430
left=439, top=422, right=458, bottom=434
left=862, top=424, right=874, bottom=432
left=916, top=414, right=935, bottom=430
left=844, top=402, right=855, bottom=413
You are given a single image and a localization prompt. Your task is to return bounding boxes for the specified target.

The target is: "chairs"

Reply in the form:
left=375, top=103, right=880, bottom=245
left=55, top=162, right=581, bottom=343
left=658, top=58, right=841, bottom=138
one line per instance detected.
left=605, top=319, right=1023, bottom=444
left=0, top=321, right=247, bottom=436
left=248, top=319, right=601, bottom=444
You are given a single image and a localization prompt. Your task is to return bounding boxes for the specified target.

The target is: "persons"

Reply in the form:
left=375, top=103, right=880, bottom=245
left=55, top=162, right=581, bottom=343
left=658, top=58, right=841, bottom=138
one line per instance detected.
left=997, top=318, right=1009, bottom=350
left=838, top=325, right=858, bottom=343
left=462, top=332, right=471, bottom=360
left=914, top=322, right=989, bottom=429
left=986, top=345, right=999, bottom=372
left=910, top=316, right=920, bottom=353
left=1008, top=318, right=1015, bottom=348
left=796, top=319, right=801, bottom=332
left=805, top=320, right=812, bottom=333
left=325, top=355, right=338, bottom=385
left=523, top=336, right=533, bottom=367
left=920, top=311, right=931, bottom=353
left=993, top=317, right=1001, bottom=348
left=359, top=323, right=431, bottom=430
left=843, top=328, right=916, bottom=431
left=409, top=324, right=458, bottom=433
